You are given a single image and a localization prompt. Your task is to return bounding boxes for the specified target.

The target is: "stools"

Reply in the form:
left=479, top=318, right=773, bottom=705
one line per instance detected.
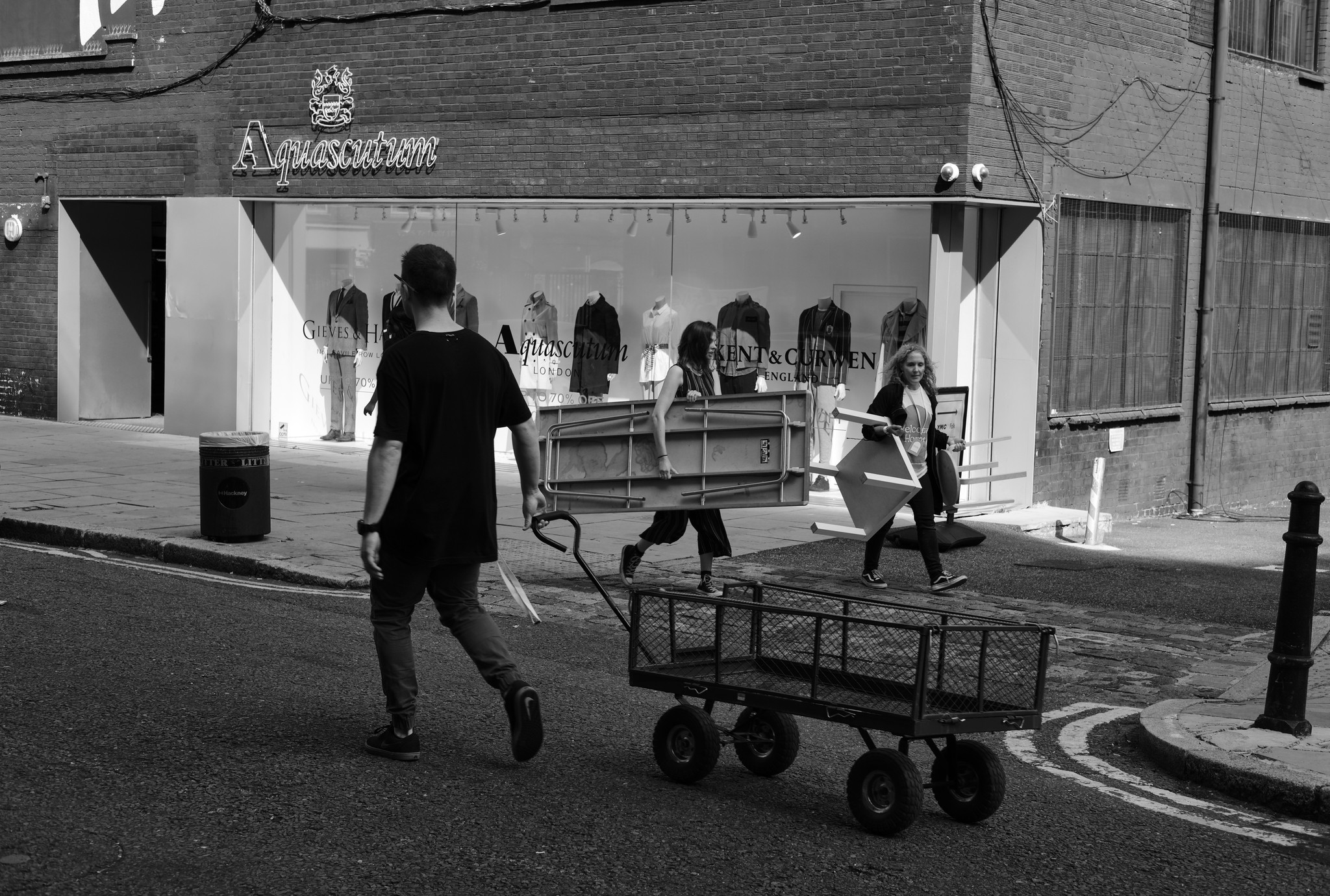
left=935, top=436, right=1026, bottom=508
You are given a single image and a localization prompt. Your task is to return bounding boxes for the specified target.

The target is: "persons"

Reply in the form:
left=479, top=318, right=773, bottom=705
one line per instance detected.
left=712, top=290, right=771, bottom=395
left=619, top=320, right=733, bottom=598
left=793, top=296, right=851, bottom=492
left=873, top=296, right=928, bottom=399
left=317, top=278, right=369, bottom=443
left=518, top=290, right=559, bottom=425
left=639, top=295, right=678, bottom=400
left=860, top=342, right=968, bottom=593
left=569, top=290, right=621, bottom=404
left=357, top=242, right=550, bottom=763
left=447, top=282, right=479, bottom=335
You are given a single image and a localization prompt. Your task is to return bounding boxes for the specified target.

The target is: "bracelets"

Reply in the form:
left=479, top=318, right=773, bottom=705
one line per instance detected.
left=885, top=426, right=892, bottom=437
left=658, top=455, right=667, bottom=459
left=883, top=426, right=889, bottom=434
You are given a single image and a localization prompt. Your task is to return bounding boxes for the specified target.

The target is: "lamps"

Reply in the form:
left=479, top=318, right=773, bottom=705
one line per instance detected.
left=802, top=208, right=809, bottom=224
left=722, top=207, right=727, bottom=223
left=838, top=208, right=847, bottom=225
left=773, top=209, right=801, bottom=238
left=684, top=208, right=691, bottom=223
left=619, top=209, right=638, bottom=237
left=354, top=207, right=447, bottom=233
left=608, top=207, right=615, bottom=223
left=736, top=209, right=758, bottom=238
left=574, top=207, right=581, bottom=223
left=657, top=209, right=673, bottom=237
left=513, top=208, right=519, bottom=222
left=485, top=208, right=506, bottom=235
left=760, top=208, right=767, bottom=223
left=647, top=208, right=653, bottom=223
left=475, top=207, right=480, bottom=221
left=541, top=207, right=548, bottom=223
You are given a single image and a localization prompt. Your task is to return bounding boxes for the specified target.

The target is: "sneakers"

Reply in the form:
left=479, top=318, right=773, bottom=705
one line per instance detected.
left=861, top=569, right=887, bottom=587
left=619, top=545, right=641, bottom=587
left=810, top=475, right=830, bottom=491
left=365, top=720, right=420, bottom=761
left=695, top=574, right=723, bottom=597
left=931, top=570, right=967, bottom=591
left=504, top=680, right=544, bottom=762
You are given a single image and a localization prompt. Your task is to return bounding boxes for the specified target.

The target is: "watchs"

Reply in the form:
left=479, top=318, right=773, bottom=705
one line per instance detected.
left=357, top=519, right=380, bottom=536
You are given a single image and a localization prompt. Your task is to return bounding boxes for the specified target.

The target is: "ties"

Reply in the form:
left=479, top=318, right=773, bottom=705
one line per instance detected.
left=336, top=288, right=345, bottom=314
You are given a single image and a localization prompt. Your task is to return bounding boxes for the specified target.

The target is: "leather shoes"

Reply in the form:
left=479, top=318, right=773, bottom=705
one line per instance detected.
left=320, top=429, right=341, bottom=440
left=336, top=432, right=356, bottom=441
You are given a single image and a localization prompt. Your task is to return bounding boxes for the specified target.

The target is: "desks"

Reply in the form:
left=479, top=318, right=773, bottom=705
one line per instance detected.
left=809, top=408, right=922, bottom=541
left=534, top=389, right=812, bottom=514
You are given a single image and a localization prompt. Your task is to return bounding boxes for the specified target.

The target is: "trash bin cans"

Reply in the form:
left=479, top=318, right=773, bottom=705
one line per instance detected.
left=199, top=431, right=270, bottom=543
